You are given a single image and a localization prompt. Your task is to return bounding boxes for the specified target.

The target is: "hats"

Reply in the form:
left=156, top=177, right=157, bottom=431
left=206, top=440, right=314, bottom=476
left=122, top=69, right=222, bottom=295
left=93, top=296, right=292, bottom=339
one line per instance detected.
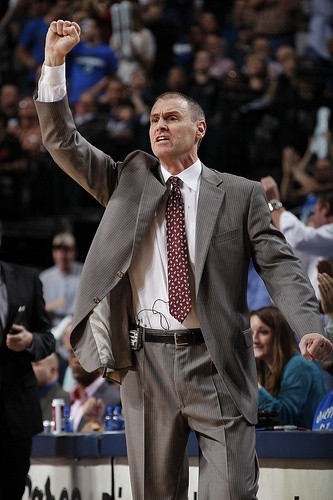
left=52, top=231, right=75, bottom=249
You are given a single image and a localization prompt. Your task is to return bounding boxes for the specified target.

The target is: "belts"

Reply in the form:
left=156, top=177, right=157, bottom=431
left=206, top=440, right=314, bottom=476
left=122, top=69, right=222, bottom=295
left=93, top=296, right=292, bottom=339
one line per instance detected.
left=145, top=329, right=205, bottom=346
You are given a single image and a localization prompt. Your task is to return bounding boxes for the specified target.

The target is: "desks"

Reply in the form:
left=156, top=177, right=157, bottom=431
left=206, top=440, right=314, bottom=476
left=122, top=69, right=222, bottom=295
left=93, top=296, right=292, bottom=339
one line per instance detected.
left=21, top=431, right=333, bottom=500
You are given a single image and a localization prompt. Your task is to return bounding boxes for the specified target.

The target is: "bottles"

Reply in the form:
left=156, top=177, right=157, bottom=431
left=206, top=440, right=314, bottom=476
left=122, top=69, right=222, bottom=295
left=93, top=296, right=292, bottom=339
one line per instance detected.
left=63, top=407, right=74, bottom=433
left=51, top=399, right=65, bottom=434
left=104, top=406, right=125, bottom=432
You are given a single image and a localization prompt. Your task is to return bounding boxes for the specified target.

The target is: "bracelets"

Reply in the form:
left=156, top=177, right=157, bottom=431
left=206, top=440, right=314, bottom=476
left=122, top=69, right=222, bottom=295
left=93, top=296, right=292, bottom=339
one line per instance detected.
left=267, top=199, right=281, bottom=212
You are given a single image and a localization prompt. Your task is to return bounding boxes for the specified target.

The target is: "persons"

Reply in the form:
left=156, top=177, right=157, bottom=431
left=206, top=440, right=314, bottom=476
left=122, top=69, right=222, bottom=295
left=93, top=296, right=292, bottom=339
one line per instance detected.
left=0, top=260, right=55, bottom=500
left=246, top=137, right=333, bottom=344
left=250, top=306, right=333, bottom=430
left=33, top=19, right=333, bottom=500
left=0, top=0, right=333, bottom=152
left=27, top=233, right=124, bottom=433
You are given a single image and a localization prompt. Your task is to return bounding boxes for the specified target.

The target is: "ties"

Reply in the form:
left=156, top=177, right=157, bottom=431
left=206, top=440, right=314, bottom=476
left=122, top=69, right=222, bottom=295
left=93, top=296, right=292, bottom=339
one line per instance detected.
left=164, top=176, right=192, bottom=325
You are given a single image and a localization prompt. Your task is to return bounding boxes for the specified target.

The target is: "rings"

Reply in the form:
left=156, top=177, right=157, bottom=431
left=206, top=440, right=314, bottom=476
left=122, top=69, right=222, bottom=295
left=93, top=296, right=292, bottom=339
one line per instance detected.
left=320, top=345, right=326, bottom=351
left=325, top=289, right=329, bottom=292
